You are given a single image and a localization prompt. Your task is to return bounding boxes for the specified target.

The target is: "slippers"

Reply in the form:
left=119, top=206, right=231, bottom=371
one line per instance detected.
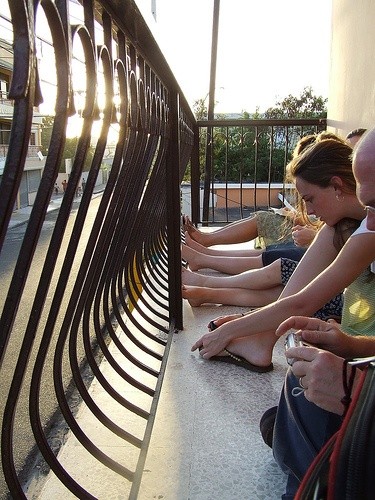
left=181, top=213, right=209, bottom=247
left=199, top=320, right=273, bottom=373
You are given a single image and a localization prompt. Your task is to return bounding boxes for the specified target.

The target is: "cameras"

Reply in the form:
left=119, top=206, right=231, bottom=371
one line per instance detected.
left=284, top=332, right=319, bottom=365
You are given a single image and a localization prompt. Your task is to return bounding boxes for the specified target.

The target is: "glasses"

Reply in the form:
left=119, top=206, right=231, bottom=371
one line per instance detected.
left=363, top=205, right=375, bottom=217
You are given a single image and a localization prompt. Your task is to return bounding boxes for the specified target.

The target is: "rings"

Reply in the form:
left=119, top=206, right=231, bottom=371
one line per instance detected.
left=300, top=377, right=304, bottom=389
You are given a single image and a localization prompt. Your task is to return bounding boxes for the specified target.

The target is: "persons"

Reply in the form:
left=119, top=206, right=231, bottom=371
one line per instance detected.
left=55, top=177, right=87, bottom=199
left=259, top=128, right=375, bottom=499
left=183, top=134, right=323, bottom=249
left=180, top=256, right=345, bottom=317
left=181, top=128, right=370, bottom=275
left=192, top=139, right=375, bottom=372
